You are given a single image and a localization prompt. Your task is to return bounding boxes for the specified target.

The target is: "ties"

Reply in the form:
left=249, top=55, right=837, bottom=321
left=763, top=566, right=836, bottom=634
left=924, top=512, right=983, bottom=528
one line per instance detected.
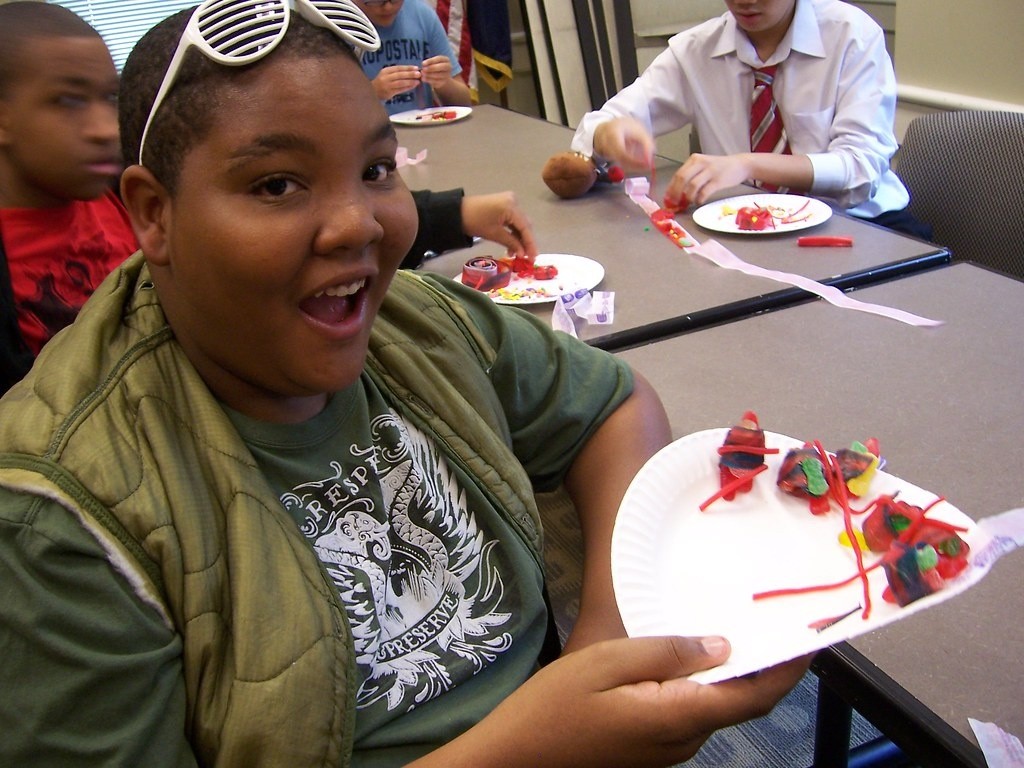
left=751, top=64, right=804, bottom=197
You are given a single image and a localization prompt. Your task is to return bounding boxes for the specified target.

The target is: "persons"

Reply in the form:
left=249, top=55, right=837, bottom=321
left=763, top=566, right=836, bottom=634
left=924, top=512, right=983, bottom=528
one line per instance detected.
left=0, top=0, right=536, bottom=396
left=570, top=0, right=933, bottom=243
left=345, top=1, right=472, bottom=116
left=0, top=1, right=817, bottom=768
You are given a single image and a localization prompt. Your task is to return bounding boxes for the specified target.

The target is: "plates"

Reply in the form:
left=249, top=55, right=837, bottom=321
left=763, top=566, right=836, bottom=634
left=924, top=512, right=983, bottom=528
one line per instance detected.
left=692, top=194, right=833, bottom=234
left=452, top=253, right=605, bottom=305
left=389, top=106, right=472, bottom=126
left=611, top=427, right=998, bottom=686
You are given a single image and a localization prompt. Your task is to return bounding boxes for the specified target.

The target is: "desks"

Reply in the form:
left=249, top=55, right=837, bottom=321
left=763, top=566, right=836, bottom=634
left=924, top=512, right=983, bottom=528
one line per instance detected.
left=393, top=101, right=955, bottom=349
left=608, top=259, right=1024, bottom=768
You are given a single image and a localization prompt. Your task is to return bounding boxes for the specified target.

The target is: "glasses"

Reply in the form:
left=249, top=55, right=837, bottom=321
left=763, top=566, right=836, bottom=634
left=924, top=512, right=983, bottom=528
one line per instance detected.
left=138, top=0, right=381, bottom=165
left=363, top=0, right=399, bottom=7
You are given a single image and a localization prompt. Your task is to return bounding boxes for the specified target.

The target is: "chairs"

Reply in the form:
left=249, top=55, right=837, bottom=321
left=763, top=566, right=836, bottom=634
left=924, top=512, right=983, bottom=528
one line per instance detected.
left=895, top=109, right=1023, bottom=279
